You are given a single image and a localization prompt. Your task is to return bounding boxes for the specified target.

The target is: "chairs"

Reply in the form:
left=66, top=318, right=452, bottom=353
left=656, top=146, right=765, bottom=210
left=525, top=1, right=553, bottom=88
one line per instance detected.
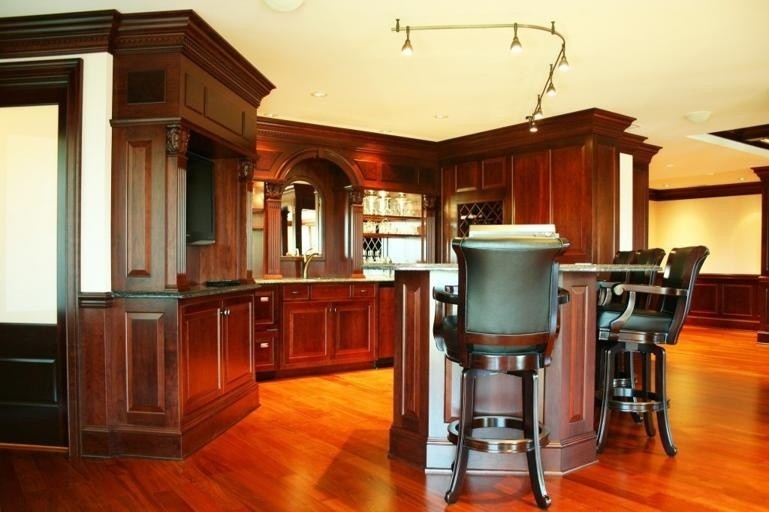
left=434, top=229, right=574, bottom=508
left=596, top=243, right=713, bottom=455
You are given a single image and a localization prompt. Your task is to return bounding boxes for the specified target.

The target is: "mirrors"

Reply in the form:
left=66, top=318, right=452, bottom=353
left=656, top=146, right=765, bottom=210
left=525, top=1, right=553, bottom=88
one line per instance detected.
left=280, top=174, right=328, bottom=263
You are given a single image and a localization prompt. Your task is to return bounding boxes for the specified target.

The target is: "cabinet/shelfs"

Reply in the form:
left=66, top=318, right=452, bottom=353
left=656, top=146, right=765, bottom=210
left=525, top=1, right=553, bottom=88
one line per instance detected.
left=362, top=190, right=425, bottom=267
left=376, top=281, right=397, bottom=367
left=252, top=290, right=275, bottom=372
left=279, top=280, right=378, bottom=368
left=103, top=286, right=261, bottom=469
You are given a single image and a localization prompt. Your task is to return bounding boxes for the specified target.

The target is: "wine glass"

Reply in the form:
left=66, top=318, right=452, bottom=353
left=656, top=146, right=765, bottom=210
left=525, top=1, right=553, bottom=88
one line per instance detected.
left=364, top=193, right=416, bottom=216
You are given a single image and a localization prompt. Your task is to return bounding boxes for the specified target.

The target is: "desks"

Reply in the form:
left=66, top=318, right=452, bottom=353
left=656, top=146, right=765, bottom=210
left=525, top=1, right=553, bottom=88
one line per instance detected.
left=387, top=263, right=663, bottom=477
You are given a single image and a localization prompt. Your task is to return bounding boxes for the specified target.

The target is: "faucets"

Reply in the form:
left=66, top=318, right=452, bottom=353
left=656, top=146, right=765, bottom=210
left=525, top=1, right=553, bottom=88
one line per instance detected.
left=285, top=248, right=300, bottom=257
left=303, top=247, right=319, bottom=278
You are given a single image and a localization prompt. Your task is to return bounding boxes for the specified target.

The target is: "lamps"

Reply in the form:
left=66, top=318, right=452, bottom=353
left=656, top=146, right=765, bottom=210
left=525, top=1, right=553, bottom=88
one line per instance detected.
left=393, top=20, right=571, bottom=139
